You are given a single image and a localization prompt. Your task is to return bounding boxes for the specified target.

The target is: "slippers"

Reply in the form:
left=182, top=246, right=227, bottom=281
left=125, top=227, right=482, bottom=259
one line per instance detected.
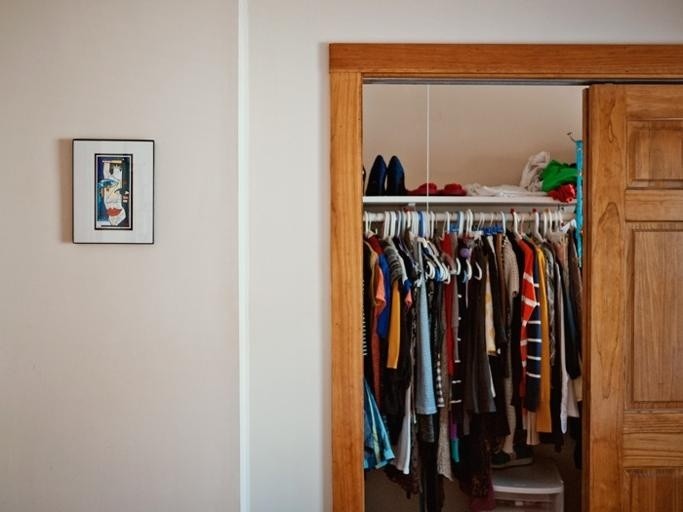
left=405, top=182, right=467, bottom=196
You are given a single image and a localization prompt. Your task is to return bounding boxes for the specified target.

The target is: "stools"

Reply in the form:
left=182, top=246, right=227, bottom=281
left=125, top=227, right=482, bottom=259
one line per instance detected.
left=490, top=459, right=565, bottom=511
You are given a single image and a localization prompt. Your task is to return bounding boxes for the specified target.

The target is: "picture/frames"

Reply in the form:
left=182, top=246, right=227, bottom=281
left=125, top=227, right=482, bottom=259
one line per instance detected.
left=72, top=138, right=154, bottom=245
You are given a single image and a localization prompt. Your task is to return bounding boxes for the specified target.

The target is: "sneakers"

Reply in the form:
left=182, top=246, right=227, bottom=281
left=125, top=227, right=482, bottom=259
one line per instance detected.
left=489, top=446, right=533, bottom=468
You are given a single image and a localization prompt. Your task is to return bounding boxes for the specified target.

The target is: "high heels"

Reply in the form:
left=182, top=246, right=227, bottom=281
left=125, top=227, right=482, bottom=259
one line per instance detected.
left=366, top=155, right=405, bottom=196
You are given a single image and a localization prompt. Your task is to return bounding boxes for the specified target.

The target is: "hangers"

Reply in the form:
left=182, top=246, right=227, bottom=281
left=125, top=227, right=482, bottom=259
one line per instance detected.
left=363, top=204, right=578, bottom=285
